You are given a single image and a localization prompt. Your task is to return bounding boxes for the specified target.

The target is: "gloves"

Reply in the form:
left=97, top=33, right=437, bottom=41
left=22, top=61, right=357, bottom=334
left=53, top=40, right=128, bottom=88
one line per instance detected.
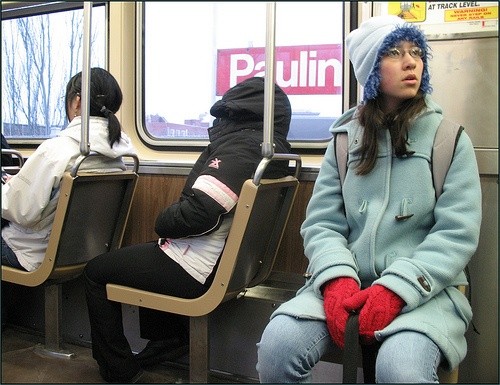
left=342, top=284, right=405, bottom=345
left=323, top=278, right=362, bottom=351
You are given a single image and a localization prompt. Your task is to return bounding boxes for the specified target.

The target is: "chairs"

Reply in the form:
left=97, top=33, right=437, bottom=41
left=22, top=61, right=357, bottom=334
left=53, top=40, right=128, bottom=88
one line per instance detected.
left=106, top=154, right=302, bottom=385
left=0, top=151, right=139, bottom=361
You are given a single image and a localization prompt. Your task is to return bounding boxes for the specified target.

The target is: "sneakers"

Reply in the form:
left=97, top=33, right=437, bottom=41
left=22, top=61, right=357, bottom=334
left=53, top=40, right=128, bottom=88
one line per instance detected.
left=134, top=332, right=189, bottom=369
left=112, top=369, right=145, bottom=383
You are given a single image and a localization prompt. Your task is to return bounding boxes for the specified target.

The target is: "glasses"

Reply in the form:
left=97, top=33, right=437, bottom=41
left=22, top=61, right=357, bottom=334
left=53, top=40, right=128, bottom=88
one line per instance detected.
left=383, top=46, right=427, bottom=58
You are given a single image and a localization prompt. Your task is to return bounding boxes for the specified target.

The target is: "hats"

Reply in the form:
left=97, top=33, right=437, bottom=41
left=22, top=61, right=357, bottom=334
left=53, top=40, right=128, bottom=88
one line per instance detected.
left=345, top=15, right=434, bottom=101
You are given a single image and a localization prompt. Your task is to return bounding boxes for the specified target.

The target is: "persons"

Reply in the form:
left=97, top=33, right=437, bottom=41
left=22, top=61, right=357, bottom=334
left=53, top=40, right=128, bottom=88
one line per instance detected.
left=1, top=68, right=130, bottom=271
left=83, top=77, right=291, bottom=384
left=256, top=14, right=483, bottom=385
